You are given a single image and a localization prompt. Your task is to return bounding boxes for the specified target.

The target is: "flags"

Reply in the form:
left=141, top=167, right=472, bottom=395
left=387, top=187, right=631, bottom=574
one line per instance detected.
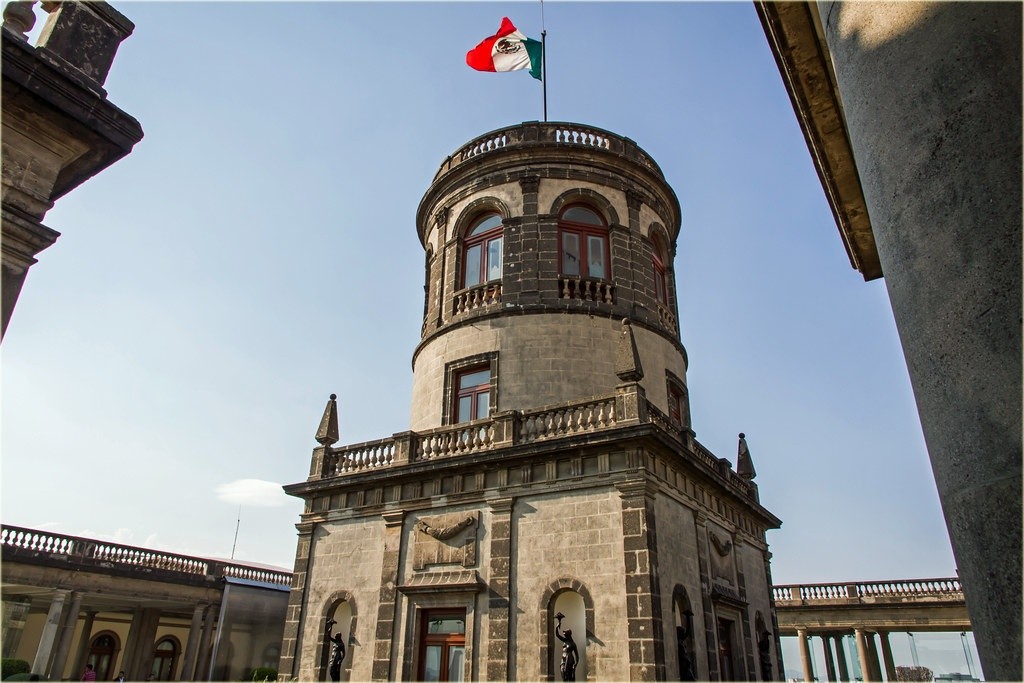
left=466, top=17, right=542, bottom=83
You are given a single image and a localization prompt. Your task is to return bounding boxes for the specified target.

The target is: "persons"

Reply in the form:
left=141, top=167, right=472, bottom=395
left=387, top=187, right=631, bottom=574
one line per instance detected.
left=113, top=671, right=126, bottom=683
left=328, top=626, right=346, bottom=683
left=555, top=623, right=579, bottom=683
left=80, top=664, right=96, bottom=683
left=145, top=674, right=155, bottom=683
